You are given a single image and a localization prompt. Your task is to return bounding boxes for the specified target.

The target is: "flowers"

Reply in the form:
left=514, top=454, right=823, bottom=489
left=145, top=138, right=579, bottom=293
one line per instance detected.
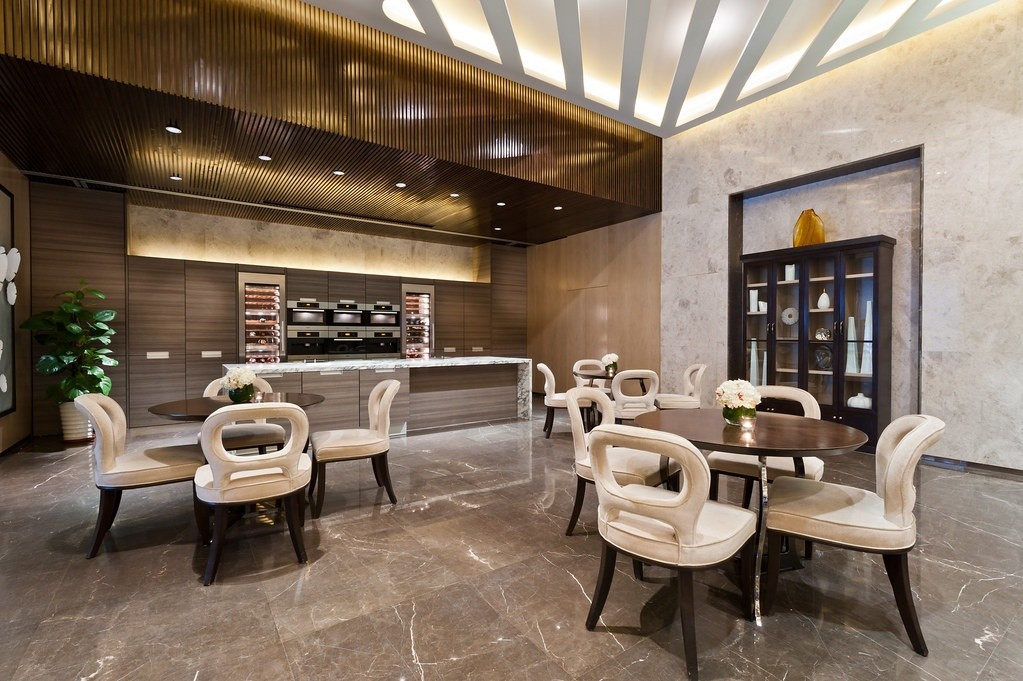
left=713, top=379, right=762, bottom=410
left=221, top=368, right=258, bottom=390
left=601, top=353, right=620, bottom=366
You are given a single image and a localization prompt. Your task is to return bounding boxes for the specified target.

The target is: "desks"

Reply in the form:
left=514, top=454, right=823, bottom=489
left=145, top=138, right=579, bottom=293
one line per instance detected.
left=572, top=368, right=651, bottom=428
left=635, top=407, right=868, bottom=576
left=149, top=392, right=324, bottom=512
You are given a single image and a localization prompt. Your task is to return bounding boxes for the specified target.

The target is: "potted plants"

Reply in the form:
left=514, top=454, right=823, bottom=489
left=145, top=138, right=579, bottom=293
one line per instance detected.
left=20, top=288, right=118, bottom=440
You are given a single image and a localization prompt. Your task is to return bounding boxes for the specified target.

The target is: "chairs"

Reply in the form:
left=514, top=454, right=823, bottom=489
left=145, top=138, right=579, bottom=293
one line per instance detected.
left=598, top=370, right=660, bottom=425
left=565, top=387, right=681, bottom=535
left=308, top=379, right=401, bottom=518
left=764, top=412, right=946, bottom=656
left=203, top=377, right=273, bottom=397
left=705, top=385, right=824, bottom=560
left=193, top=401, right=309, bottom=586
left=572, top=360, right=612, bottom=425
left=73, top=392, right=208, bottom=559
left=585, top=425, right=757, bottom=681
left=654, top=364, right=706, bottom=409
left=537, top=363, right=593, bottom=439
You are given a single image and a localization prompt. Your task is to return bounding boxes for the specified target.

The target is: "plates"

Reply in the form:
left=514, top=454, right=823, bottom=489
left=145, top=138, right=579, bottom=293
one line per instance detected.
left=813, top=345, right=833, bottom=369
left=815, top=328, right=831, bottom=340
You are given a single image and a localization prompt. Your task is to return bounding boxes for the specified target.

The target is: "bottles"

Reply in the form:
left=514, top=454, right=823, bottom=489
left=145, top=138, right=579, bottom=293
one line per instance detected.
left=817, top=292, right=830, bottom=309
left=847, top=392, right=872, bottom=410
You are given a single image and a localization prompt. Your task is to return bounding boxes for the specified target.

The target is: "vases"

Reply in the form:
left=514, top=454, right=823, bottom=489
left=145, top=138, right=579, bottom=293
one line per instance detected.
left=229, top=384, right=254, bottom=402
left=723, top=406, right=757, bottom=425
left=605, top=363, right=617, bottom=376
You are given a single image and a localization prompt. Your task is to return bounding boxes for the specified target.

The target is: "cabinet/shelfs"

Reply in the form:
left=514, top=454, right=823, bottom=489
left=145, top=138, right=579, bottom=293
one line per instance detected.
left=740, top=234, right=897, bottom=454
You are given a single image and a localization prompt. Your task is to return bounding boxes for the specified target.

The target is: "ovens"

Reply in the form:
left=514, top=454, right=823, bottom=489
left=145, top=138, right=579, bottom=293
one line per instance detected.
left=286, top=299, right=401, bottom=361
